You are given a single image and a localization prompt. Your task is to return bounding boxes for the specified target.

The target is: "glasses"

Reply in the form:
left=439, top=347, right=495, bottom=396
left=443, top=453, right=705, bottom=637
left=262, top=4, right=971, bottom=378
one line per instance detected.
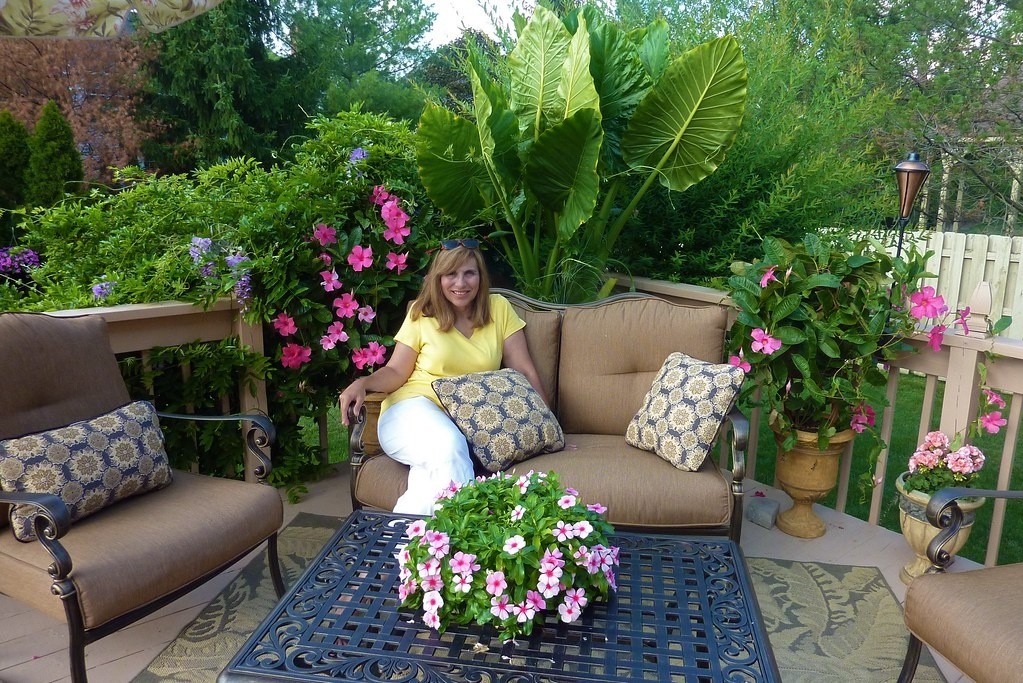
left=439, top=240, right=479, bottom=250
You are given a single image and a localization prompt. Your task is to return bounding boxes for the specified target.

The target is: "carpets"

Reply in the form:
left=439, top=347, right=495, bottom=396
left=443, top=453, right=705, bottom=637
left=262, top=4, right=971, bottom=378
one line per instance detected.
left=126, top=512, right=952, bottom=683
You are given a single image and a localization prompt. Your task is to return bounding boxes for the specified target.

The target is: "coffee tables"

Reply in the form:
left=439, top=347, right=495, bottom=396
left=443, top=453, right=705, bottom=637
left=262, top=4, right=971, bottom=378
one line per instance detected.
left=216, top=509, right=783, bottom=683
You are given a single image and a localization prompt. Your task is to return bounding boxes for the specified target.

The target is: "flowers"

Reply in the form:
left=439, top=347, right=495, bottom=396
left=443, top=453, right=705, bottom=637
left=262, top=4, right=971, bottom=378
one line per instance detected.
left=202, top=162, right=436, bottom=422
left=399, top=472, right=617, bottom=646
left=723, top=230, right=1008, bottom=503
left=901, top=430, right=985, bottom=502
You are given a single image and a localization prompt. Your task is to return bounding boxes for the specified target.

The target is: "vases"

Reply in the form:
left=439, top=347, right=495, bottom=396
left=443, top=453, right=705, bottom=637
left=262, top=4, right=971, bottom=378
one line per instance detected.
left=775, top=412, right=855, bottom=539
left=895, top=472, right=986, bottom=584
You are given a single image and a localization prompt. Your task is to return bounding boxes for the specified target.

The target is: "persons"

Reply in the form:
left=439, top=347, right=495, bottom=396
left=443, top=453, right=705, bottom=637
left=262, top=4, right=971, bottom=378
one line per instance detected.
left=325, top=238, right=577, bottom=658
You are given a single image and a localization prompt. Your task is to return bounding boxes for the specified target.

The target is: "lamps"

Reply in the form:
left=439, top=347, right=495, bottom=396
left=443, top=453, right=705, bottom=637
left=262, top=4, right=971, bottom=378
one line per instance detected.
left=871, top=153, right=932, bottom=359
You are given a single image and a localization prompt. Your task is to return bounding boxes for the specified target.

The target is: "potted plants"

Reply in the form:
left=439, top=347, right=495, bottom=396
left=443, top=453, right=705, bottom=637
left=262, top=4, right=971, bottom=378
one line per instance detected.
left=342, top=388, right=383, bottom=457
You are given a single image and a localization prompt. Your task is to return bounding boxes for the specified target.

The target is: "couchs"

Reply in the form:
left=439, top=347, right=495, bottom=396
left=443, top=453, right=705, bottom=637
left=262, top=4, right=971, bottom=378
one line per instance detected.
left=348, top=287, right=746, bottom=631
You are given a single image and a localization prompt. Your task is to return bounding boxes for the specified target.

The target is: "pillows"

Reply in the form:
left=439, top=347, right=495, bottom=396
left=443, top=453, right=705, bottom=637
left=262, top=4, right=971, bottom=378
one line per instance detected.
left=0, top=398, right=173, bottom=541
left=626, top=351, right=745, bottom=472
left=430, top=367, right=566, bottom=475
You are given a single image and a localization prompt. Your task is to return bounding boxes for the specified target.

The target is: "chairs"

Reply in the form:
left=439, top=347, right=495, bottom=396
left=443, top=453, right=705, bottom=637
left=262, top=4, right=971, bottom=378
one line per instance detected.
left=895, top=487, right=1023, bottom=683
left=1, top=311, right=287, bottom=683
